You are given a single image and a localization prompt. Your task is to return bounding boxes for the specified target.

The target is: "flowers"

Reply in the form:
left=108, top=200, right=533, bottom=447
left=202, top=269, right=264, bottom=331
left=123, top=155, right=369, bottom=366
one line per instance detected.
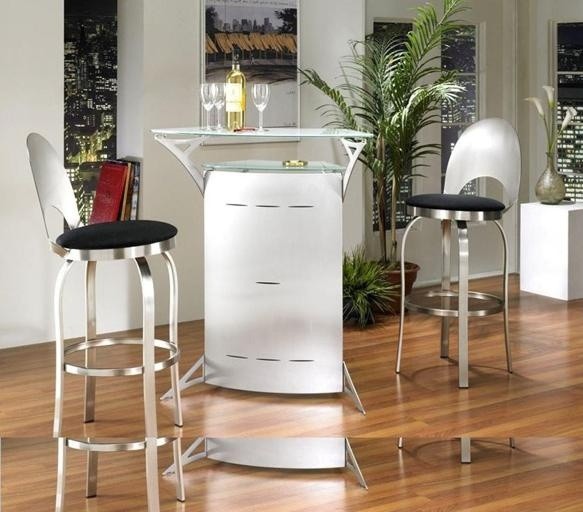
left=523, top=82, right=577, bottom=152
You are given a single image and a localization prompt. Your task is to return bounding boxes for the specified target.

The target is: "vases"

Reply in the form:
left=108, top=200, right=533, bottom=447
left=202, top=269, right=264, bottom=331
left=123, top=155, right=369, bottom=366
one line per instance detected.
left=534, top=153, right=566, bottom=205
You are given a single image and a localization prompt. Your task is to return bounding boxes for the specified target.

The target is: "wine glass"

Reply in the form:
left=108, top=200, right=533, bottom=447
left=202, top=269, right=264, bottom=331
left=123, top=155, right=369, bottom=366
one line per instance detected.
left=211, top=82, right=229, bottom=133
left=250, top=82, right=271, bottom=134
left=200, top=82, right=219, bottom=133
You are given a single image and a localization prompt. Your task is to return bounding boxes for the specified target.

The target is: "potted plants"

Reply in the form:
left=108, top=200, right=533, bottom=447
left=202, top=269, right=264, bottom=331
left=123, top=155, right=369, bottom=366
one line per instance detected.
left=292, top=0, right=474, bottom=313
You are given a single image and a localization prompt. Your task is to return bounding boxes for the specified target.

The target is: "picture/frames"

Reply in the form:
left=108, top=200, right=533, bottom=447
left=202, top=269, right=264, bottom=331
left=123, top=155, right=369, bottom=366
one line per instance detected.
left=198, top=1, right=300, bottom=146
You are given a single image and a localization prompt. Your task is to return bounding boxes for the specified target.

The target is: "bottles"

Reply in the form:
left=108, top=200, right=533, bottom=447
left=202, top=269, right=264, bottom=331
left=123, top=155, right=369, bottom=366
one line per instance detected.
left=225, top=60, right=247, bottom=132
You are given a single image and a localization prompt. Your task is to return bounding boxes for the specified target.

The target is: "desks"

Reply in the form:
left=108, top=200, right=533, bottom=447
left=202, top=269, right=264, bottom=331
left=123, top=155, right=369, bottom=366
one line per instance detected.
left=148, top=127, right=373, bottom=415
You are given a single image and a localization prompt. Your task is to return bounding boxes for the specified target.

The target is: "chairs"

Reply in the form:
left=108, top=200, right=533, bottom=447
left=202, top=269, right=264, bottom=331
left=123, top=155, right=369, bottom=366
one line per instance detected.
left=394, top=116, right=523, bottom=389
left=26, top=132, right=183, bottom=432
